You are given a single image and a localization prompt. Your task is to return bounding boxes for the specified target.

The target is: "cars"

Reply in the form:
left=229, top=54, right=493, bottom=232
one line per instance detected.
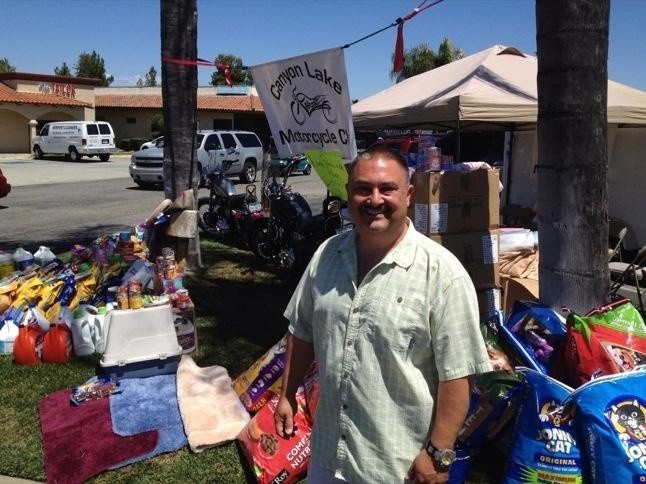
left=139, top=134, right=166, bottom=150
left=268, top=151, right=312, bottom=176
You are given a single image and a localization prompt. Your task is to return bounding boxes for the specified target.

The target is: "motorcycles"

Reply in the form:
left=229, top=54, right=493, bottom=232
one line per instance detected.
left=191, top=146, right=264, bottom=244
left=242, top=153, right=346, bottom=282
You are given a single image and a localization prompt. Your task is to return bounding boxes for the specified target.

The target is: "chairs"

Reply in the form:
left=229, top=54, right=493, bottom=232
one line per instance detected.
left=608, top=226, right=646, bottom=314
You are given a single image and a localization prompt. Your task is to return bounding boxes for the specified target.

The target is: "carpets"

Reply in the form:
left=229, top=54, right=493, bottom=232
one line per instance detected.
left=37, top=353, right=251, bottom=483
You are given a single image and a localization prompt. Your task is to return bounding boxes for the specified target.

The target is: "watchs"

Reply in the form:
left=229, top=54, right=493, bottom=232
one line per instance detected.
left=425, top=438, right=458, bottom=468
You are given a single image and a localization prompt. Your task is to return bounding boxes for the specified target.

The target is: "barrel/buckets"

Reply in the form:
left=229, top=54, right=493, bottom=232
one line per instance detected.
left=1, top=245, right=56, bottom=279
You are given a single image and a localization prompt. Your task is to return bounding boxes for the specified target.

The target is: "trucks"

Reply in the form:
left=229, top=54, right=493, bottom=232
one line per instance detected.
left=29, top=119, right=116, bottom=163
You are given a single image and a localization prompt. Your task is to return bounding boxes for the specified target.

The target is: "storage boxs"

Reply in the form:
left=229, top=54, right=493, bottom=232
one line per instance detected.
left=411, top=164, right=502, bottom=324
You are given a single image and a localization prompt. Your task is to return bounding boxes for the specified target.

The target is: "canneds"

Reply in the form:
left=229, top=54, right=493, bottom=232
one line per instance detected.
left=155, top=246, right=190, bottom=310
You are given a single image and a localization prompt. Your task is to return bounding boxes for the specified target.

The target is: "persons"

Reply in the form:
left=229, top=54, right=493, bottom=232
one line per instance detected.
left=273, top=145, right=492, bottom=484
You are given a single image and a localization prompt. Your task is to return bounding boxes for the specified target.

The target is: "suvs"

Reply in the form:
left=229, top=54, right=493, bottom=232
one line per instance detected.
left=360, top=131, right=447, bottom=156
left=128, top=129, right=265, bottom=191
